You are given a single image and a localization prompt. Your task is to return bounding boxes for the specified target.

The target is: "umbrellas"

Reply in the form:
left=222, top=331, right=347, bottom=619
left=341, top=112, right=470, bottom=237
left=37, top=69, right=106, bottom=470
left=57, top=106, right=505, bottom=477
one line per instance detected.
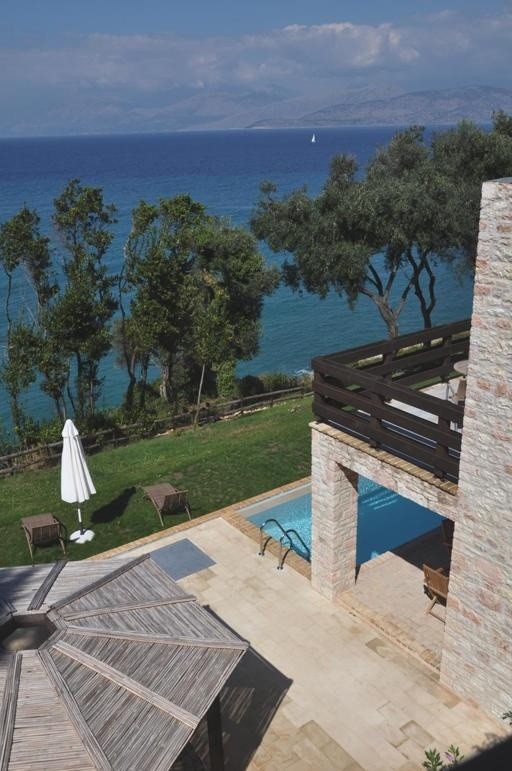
left=59, top=418, right=97, bottom=537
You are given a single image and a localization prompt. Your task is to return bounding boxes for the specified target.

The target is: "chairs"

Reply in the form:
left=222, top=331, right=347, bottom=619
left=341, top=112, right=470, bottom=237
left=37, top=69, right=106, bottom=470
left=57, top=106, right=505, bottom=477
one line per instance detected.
left=445, top=378, right=466, bottom=431
left=139, top=483, right=192, bottom=527
left=421, top=519, right=457, bottom=617
left=20, top=511, right=66, bottom=560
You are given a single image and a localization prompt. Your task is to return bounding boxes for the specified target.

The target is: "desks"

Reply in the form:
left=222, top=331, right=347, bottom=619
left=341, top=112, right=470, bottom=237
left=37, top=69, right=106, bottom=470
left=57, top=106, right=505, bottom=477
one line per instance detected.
left=453, top=360, right=468, bottom=379
